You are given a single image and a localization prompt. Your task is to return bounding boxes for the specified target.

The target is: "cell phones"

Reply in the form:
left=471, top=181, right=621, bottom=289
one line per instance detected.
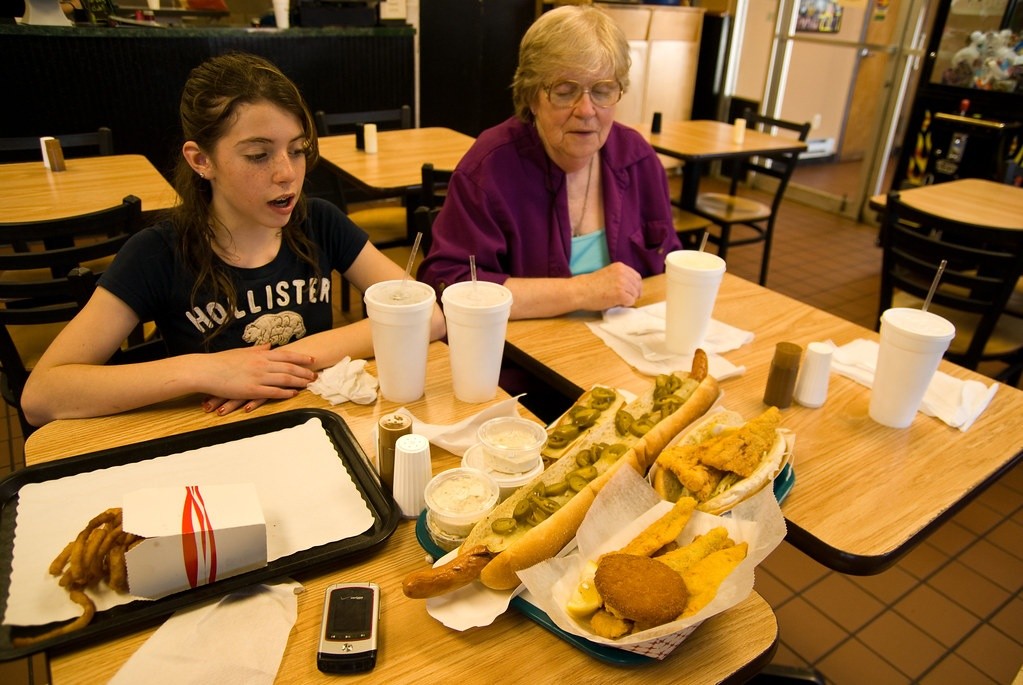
left=318, top=581, right=380, bottom=674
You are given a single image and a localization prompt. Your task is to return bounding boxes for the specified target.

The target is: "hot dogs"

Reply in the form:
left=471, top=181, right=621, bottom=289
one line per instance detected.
left=654, top=406, right=787, bottom=516
left=398, top=347, right=721, bottom=599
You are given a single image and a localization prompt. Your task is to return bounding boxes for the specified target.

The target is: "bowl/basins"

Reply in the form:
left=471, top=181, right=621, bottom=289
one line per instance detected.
left=424, top=416, right=548, bottom=552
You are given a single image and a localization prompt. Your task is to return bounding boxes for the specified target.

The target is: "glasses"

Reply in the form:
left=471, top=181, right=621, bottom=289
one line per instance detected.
left=537, top=74, right=625, bottom=109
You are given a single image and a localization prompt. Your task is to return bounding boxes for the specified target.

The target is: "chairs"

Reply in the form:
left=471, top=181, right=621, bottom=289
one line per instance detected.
left=315, top=104, right=435, bottom=315
left=670, top=107, right=812, bottom=286
left=411, top=205, right=444, bottom=259
left=0, top=126, right=115, bottom=252
left=875, top=192, right=1023, bottom=386
left=420, top=164, right=454, bottom=216
left=665, top=202, right=711, bottom=250
left=0, top=194, right=146, bottom=440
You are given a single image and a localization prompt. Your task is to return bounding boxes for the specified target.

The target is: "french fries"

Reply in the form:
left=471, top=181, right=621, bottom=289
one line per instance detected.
left=14, top=508, right=146, bottom=646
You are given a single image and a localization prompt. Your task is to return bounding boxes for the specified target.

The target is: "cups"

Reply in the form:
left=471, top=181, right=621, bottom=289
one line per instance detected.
left=868, top=307, right=956, bottom=431
left=664, top=249, right=727, bottom=356
left=363, top=279, right=437, bottom=404
left=440, top=281, right=513, bottom=403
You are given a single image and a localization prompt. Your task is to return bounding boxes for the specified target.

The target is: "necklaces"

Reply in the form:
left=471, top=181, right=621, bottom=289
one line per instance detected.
left=568, top=156, right=595, bottom=236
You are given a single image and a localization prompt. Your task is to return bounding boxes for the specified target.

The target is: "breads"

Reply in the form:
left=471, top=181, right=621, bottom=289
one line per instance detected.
left=542, top=386, right=624, bottom=460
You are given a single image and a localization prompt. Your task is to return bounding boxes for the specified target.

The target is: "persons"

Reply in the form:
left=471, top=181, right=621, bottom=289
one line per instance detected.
left=21, top=54, right=445, bottom=425
left=416, top=5, right=681, bottom=397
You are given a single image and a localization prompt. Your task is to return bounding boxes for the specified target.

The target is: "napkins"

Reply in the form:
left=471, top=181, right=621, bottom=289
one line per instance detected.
left=583, top=319, right=746, bottom=382
left=306, top=355, right=379, bottom=406
left=600, top=299, right=754, bottom=361
left=511, top=461, right=789, bottom=646
left=830, top=338, right=999, bottom=435
left=100, top=576, right=303, bottom=685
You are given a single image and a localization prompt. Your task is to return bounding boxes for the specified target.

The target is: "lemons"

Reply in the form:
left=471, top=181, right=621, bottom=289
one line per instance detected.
left=565, top=560, right=606, bottom=616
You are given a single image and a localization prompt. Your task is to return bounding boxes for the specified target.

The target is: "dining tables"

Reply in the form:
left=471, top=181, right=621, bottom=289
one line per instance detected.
left=869, top=177, right=1023, bottom=245
left=0, top=152, right=184, bottom=278
left=504, top=269, right=1023, bottom=578
left=301, top=127, right=478, bottom=317
left=18, top=338, right=778, bottom=685
left=632, top=119, right=808, bottom=252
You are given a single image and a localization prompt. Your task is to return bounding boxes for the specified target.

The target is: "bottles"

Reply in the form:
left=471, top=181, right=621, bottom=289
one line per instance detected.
left=40, top=137, right=66, bottom=172
left=393, top=433, right=434, bottom=519
left=794, top=342, right=836, bottom=409
left=763, top=342, right=802, bottom=408
left=651, top=112, right=661, bottom=133
left=733, top=118, right=746, bottom=146
left=379, top=414, right=412, bottom=491
left=355, top=124, right=378, bottom=155
